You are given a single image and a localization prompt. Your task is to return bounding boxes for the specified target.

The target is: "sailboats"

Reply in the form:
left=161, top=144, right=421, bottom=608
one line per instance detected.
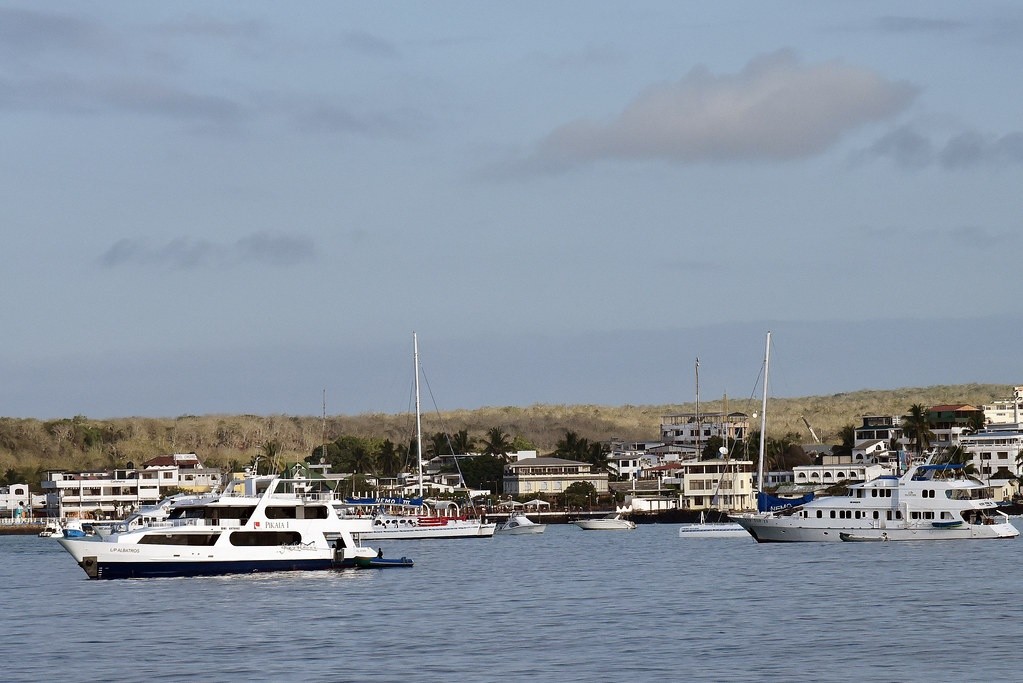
left=350, top=330, right=497, bottom=541
left=679, top=330, right=776, bottom=539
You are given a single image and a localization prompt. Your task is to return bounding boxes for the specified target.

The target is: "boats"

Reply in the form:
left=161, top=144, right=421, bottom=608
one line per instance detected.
left=574, top=518, right=638, bottom=530
left=57, top=526, right=379, bottom=581
left=728, top=457, right=1019, bottom=542
left=496, top=511, right=547, bottom=534
left=35, top=441, right=375, bottom=540
